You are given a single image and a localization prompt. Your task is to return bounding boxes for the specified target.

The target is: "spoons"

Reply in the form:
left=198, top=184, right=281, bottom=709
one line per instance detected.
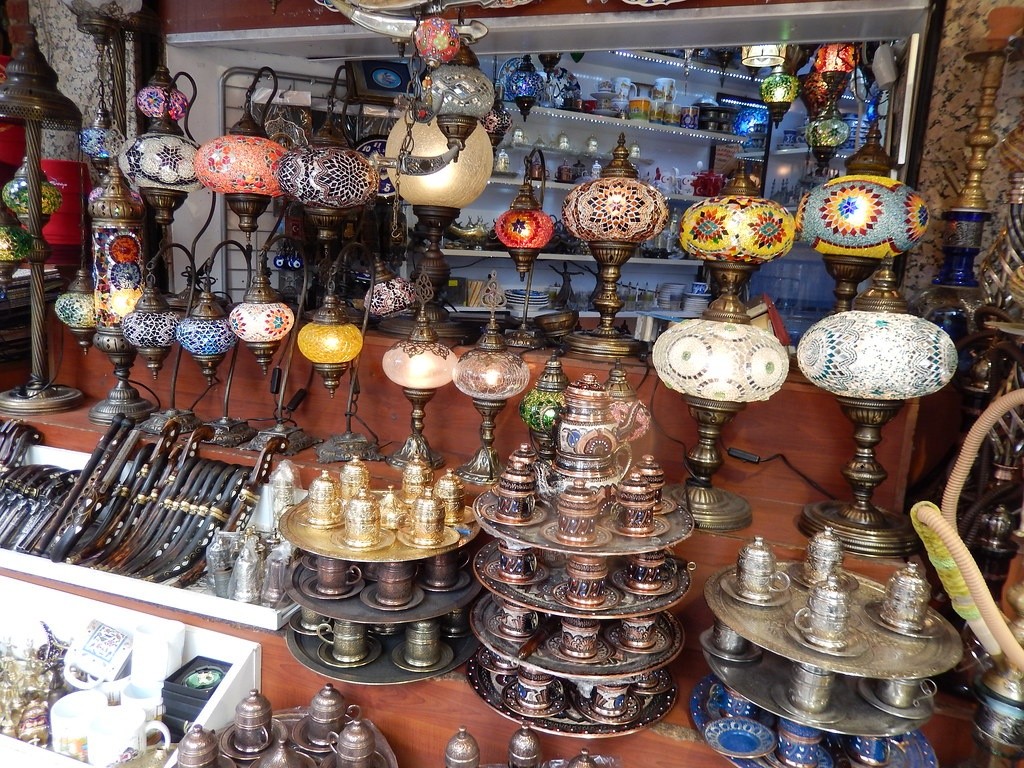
left=673, top=167, right=680, bottom=193
left=697, top=161, right=703, bottom=173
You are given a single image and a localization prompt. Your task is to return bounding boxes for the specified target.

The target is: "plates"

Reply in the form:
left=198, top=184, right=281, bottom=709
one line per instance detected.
left=484, top=613, right=612, bottom=664
left=658, top=282, right=712, bottom=314
left=506, top=289, right=549, bottom=312
left=475, top=647, right=566, bottom=718
left=786, top=601, right=946, bottom=656
left=591, top=93, right=617, bottom=99
left=765, top=744, right=833, bottom=768
left=703, top=697, right=723, bottom=718
left=747, top=132, right=765, bottom=139
left=294, top=492, right=396, bottom=552
left=591, top=109, right=617, bottom=116
left=718, top=563, right=861, bottom=609
left=483, top=559, right=678, bottom=613
left=480, top=480, right=611, bottom=550
left=841, top=739, right=910, bottom=768
left=395, top=505, right=476, bottom=548
left=776, top=142, right=809, bottom=149
left=701, top=628, right=848, bottom=724
left=390, top=631, right=471, bottom=673
left=297, top=554, right=471, bottom=612
left=745, top=146, right=765, bottom=153
left=857, top=677, right=936, bottom=720
left=558, top=106, right=583, bottom=113
left=574, top=672, right=674, bottom=725
left=492, top=170, right=576, bottom=184
left=598, top=497, right=675, bottom=538
left=171, top=714, right=390, bottom=768
left=703, top=718, right=777, bottom=759
left=287, top=614, right=382, bottom=668
left=603, top=623, right=671, bottom=654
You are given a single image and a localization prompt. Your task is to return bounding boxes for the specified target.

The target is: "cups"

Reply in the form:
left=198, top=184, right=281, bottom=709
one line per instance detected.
left=973, top=664, right=1024, bottom=757
left=308, top=553, right=472, bottom=605
left=307, top=442, right=469, bottom=547
left=747, top=122, right=766, bottom=148
left=644, top=171, right=725, bottom=197
left=735, top=525, right=931, bottom=648
left=783, top=128, right=798, bottom=143
left=49, top=619, right=374, bottom=768
left=712, top=618, right=938, bottom=717
left=495, top=538, right=675, bottom=603
left=487, top=650, right=660, bottom=718
left=296, top=605, right=464, bottom=668
left=706, top=682, right=895, bottom=768
left=595, top=77, right=733, bottom=134
left=494, top=597, right=660, bottom=660
left=492, top=443, right=667, bottom=544
left=511, top=123, right=641, bottom=158
left=691, top=282, right=709, bottom=294
left=617, top=291, right=655, bottom=313
left=494, top=149, right=585, bottom=180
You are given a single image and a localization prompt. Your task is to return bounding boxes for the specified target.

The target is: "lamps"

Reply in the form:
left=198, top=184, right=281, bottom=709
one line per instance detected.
left=0, top=0, right=957, bottom=557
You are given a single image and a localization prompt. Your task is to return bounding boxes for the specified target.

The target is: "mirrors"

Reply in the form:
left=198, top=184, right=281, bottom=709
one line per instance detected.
left=697, top=91, right=773, bottom=281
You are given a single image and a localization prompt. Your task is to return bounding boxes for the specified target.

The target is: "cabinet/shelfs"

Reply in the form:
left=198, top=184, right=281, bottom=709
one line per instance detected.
left=402, top=50, right=870, bottom=321
left=0, top=308, right=923, bottom=768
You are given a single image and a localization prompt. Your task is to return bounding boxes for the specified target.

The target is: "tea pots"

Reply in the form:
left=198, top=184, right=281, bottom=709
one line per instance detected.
left=531, top=443, right=645, bottom=510
left=539, top=373, right=646, bottom=456
